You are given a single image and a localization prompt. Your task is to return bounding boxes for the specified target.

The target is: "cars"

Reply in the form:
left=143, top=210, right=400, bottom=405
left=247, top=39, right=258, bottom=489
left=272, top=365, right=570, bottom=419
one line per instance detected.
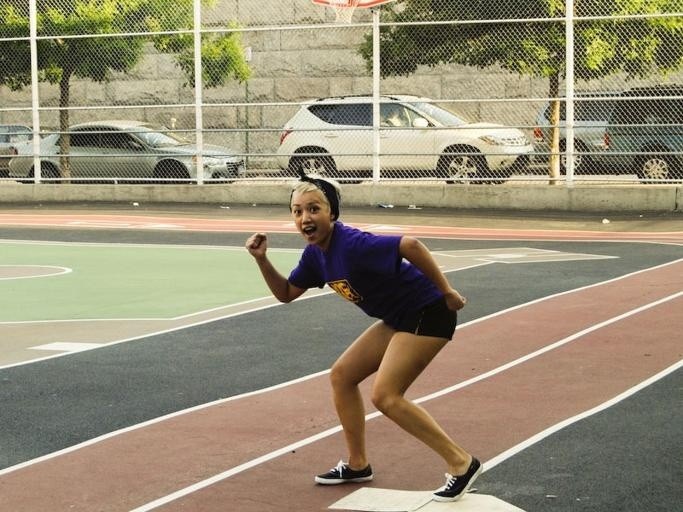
left=0, top=122, right=58, bottom=179
left=8, top=118, right=246, bottom=188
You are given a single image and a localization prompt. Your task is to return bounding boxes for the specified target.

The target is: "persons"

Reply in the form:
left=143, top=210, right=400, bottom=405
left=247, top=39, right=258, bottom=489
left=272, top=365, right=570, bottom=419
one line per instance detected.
left=246, top=174, right=483, bottom=502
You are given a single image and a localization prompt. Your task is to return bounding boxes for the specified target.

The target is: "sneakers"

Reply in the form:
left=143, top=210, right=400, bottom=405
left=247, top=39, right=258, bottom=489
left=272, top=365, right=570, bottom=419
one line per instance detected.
left=432, top=456, right=483, bottom=502
left=315, top=459, right=373, bottom=484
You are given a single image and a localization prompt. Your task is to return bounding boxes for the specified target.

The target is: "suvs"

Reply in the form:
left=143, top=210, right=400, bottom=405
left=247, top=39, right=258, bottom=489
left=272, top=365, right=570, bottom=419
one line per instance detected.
left=599, top=82, right=682, bottom=185
left=529, top=84, right=622, bottom=177
left=273, top=90, right=536, bottom=188
